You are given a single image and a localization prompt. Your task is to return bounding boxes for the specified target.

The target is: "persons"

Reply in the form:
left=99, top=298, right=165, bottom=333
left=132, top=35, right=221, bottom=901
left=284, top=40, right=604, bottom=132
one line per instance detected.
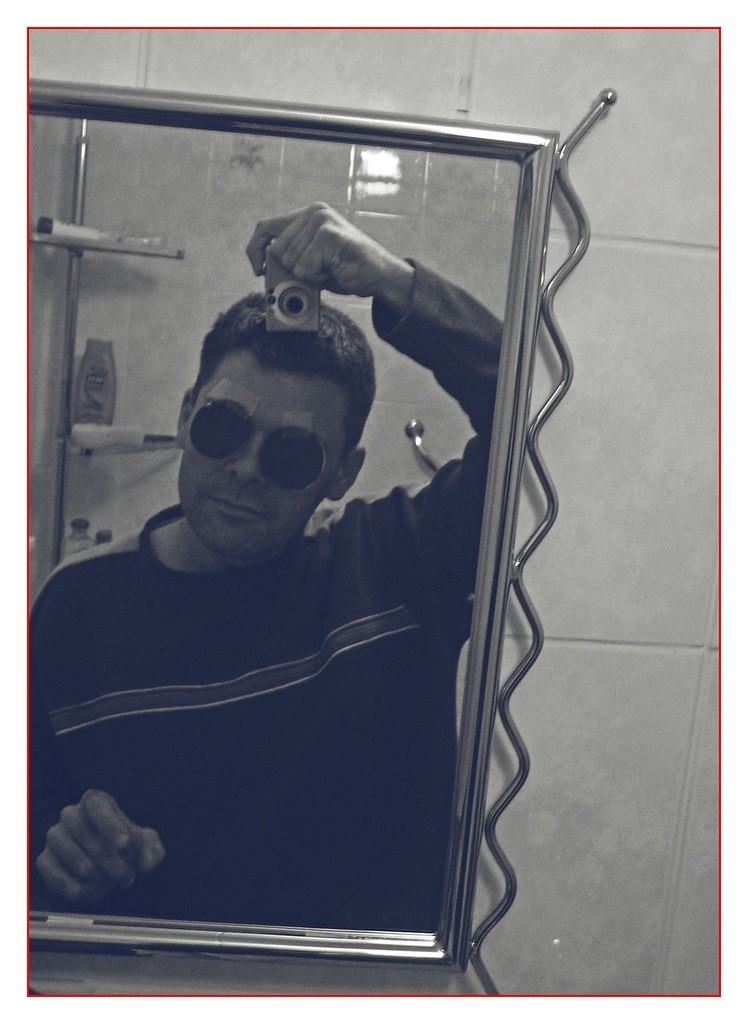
left=30, top=201, right=506, bottom=932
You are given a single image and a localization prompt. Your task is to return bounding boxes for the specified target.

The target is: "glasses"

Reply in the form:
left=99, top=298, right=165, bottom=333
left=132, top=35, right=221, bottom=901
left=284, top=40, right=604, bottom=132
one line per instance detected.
left=189, top=387, right=353, bottom=490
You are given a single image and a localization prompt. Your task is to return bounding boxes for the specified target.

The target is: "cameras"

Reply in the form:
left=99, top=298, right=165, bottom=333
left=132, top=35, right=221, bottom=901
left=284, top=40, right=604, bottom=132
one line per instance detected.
left=259, top=239, right=321, bottom=333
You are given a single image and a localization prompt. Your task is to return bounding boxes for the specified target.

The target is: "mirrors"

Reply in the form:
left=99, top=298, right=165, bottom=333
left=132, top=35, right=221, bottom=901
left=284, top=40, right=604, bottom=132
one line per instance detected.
left=26, top=76, right=616, bottom=996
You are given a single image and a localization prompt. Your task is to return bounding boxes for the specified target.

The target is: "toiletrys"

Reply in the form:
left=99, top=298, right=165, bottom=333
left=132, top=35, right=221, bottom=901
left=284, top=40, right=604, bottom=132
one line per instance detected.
left=59, top=518, right=113, bottom=563
left=36, top=215, right=111, bottom=241
left=73, top=336, right=118, bottom=427
